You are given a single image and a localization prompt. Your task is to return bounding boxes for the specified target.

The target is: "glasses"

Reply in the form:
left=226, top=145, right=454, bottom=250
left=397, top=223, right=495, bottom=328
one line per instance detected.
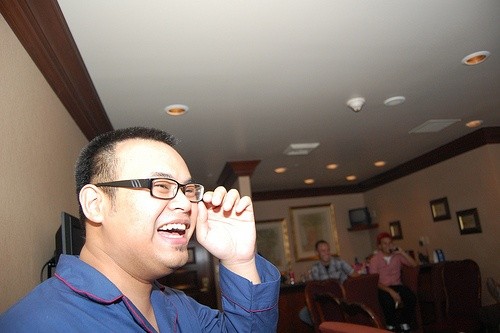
left=94, top=177, right=205, bottom=203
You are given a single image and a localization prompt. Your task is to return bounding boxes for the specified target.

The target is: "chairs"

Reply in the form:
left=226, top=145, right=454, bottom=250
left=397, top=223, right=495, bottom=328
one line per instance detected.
left=304, top=260, right=486, bottom=333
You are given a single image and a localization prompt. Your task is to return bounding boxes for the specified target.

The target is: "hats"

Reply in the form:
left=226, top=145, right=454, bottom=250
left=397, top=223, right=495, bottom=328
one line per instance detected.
left=376, top=232, right=391, bottom=245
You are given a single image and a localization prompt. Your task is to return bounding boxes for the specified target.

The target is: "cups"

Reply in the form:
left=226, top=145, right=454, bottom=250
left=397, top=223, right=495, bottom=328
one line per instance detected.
left=436, top=249, right=446, bottom=261
left=433, top=251, right=439, bottom=262
left=289, top=271, right=295, bottom=284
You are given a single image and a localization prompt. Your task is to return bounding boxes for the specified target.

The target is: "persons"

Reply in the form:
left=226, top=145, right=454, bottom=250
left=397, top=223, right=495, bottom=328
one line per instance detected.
left=299, top=240, right=359, bottom=326
left=368, top=233, right=418, bottom=333
left=0, top=126, right=281, bottom=333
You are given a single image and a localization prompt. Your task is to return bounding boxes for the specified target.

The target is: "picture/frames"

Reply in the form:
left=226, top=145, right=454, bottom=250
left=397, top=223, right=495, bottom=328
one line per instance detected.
left=389, top=222, right=402, bottom=240
left=256, top=217, right=292, bottom=274
left=456, top=208, right=482, bottom=236
left=430, top=197, right=451, bottom=222
left=289, top=202, right=340, bottom=263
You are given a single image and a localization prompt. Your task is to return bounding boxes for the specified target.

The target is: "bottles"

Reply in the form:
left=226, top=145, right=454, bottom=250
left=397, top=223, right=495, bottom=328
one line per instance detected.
left=418, top=240, right=430, bottom=266
